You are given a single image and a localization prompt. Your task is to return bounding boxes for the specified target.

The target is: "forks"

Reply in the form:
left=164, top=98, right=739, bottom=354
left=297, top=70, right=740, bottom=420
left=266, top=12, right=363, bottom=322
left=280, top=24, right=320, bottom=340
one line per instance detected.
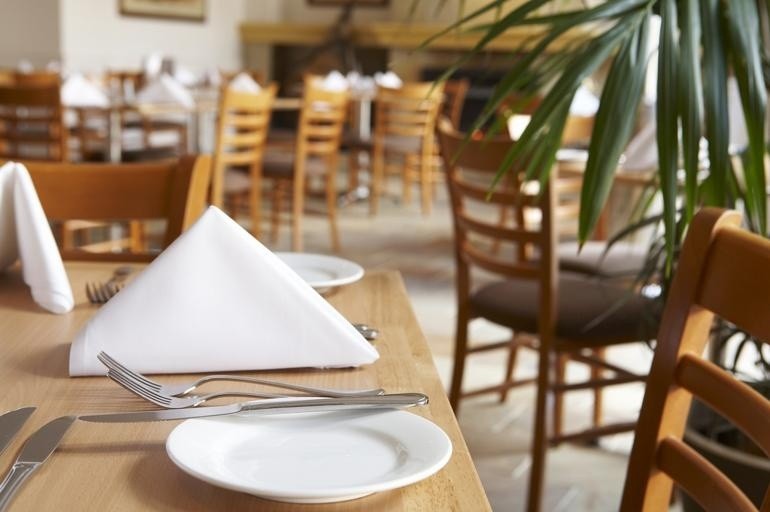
left=96, top=349, right=385, bottom=398
left=84, top=265, right=135, bottom=304
left=108, top=367, right=327, bottom=407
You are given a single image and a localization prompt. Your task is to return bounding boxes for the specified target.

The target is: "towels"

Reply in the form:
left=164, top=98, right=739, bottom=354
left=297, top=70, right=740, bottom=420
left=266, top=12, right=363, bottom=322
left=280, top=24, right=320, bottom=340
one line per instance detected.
left=1, top=158, right=76, bottom=313
left=64, top=197, right=381, bottom=375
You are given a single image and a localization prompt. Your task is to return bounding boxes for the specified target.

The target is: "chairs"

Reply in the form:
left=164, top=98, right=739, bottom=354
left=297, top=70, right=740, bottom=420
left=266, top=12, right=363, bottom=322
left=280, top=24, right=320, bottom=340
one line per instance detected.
left=618, top=206, right=770, bottom=512
left=433, top=115, right=684, bottom=512
left=1, top=48, right=472, bottom=265
left=496, top=112, right=613, bottom=450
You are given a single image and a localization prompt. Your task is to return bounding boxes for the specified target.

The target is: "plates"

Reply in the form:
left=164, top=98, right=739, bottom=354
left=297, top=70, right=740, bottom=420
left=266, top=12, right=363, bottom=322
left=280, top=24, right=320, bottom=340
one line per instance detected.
left=271, top=250, right=366, bottom=296
left=166, top=399, right=456, bottom=506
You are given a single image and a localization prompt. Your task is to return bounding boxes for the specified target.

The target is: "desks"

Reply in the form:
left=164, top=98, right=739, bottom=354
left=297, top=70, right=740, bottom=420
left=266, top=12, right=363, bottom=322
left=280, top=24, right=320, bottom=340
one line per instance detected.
left=613, top=163, right=770, bottom=381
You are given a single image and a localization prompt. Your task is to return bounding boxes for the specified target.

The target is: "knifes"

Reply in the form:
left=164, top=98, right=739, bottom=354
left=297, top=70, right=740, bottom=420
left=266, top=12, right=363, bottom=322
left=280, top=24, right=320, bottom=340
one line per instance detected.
left=0, top=416, right=76, bottom=511
left=0, top=405, right=35, bottom=456
left=76, top=393, right=431, bottom=423
